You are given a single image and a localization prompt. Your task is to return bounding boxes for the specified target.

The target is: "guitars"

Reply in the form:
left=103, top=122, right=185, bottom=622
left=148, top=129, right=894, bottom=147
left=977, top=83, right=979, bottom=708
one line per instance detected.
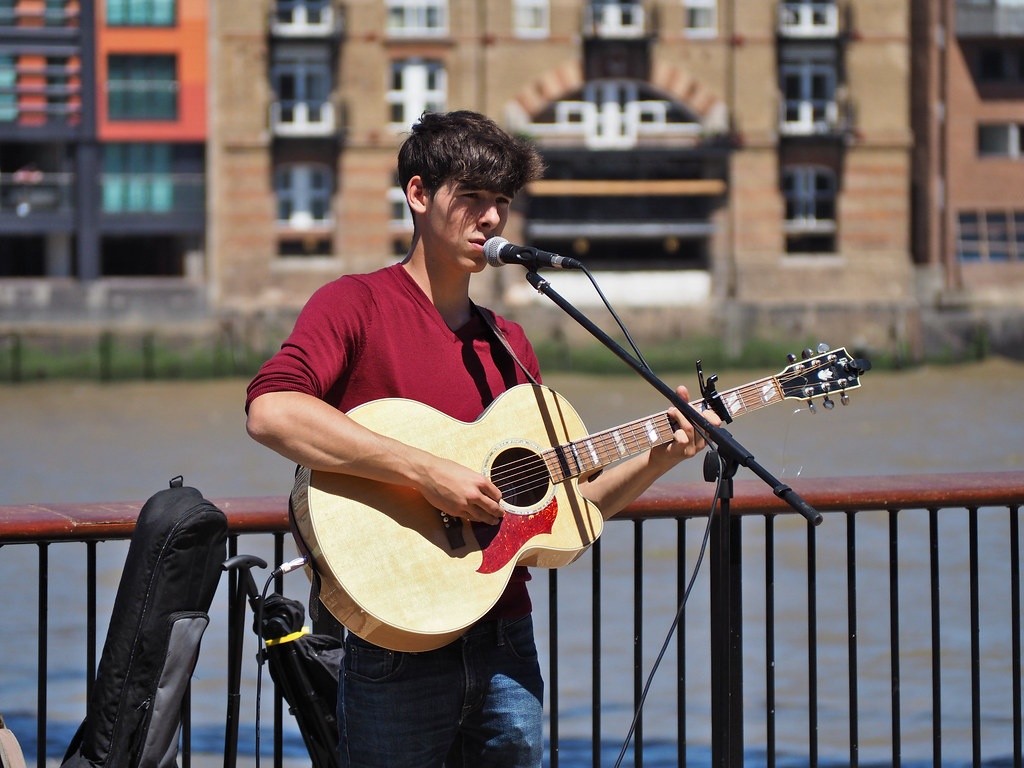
left=286, top=340, right=871, bottom=658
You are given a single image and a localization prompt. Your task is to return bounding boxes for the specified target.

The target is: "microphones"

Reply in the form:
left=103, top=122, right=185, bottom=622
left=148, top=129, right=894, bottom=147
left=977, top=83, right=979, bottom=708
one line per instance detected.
left=482, top=236, right=582, bottom=269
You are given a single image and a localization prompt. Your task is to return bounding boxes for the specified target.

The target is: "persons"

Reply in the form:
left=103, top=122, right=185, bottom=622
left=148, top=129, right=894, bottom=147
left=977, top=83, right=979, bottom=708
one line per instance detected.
left=244, top=110, right=722, bottom=768
